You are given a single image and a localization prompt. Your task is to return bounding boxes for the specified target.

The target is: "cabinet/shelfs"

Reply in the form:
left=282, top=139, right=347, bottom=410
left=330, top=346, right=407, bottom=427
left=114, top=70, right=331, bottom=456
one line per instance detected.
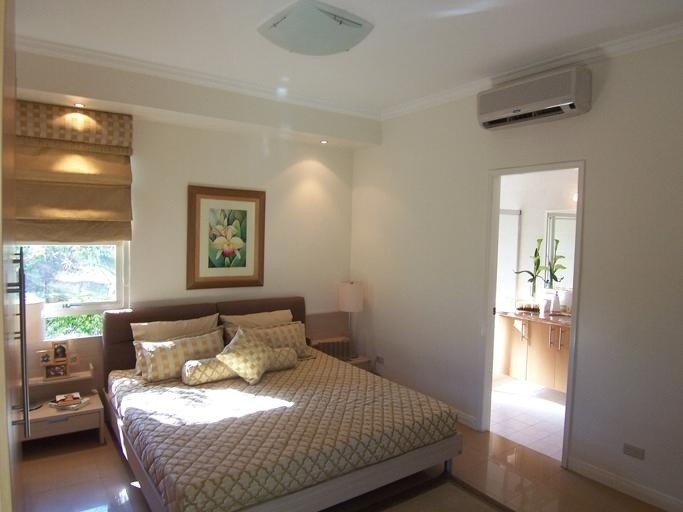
left=501, top=316, right=572, bottom=395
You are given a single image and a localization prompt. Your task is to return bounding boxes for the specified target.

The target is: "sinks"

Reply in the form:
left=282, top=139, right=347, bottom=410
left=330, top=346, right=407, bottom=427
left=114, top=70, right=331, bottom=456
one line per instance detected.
left=549, top=315, right=572, bottom=321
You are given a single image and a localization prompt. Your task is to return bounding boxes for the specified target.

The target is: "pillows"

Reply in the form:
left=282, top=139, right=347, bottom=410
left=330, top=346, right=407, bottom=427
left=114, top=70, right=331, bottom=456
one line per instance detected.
left=128, top=308, right=312, bottom=386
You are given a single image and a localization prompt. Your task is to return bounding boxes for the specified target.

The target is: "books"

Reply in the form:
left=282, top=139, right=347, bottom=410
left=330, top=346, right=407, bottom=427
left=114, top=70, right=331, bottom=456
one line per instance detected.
left=48, top=396, right=89, bottom=411
left=55, top=391, right=81, bottom=407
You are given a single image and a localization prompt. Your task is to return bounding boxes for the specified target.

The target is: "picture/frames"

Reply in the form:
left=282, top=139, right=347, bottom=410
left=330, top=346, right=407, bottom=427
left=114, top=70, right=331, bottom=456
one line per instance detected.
left=187, top=183, right=266, bottom=290
left=39, top=340, right=71, bottom=381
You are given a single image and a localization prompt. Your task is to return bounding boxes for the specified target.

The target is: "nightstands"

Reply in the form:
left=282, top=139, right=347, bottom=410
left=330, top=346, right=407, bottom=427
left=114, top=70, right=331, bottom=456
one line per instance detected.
left=19, top=387, right=106, bottom=447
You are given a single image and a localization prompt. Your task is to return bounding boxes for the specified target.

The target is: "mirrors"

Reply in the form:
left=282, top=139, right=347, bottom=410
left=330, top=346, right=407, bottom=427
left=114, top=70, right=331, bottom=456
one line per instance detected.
left=544, top=211, right=576, bottom=293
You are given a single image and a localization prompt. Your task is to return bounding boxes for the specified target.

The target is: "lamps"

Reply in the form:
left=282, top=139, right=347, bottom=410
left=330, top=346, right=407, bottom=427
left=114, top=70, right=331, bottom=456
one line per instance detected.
left=255, top=1, right=371, bottom=57
left=340, top=281, right=364, bottom=336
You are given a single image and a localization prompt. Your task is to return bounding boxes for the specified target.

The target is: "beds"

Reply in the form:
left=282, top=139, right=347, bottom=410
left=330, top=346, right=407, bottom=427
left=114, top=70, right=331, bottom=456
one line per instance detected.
left=98, top=296, right=464, bottom=512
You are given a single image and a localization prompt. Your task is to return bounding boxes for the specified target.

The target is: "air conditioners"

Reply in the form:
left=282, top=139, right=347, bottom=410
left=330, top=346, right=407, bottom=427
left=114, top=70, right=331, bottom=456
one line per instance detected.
left=475, top=64, right=592, bottom=129
